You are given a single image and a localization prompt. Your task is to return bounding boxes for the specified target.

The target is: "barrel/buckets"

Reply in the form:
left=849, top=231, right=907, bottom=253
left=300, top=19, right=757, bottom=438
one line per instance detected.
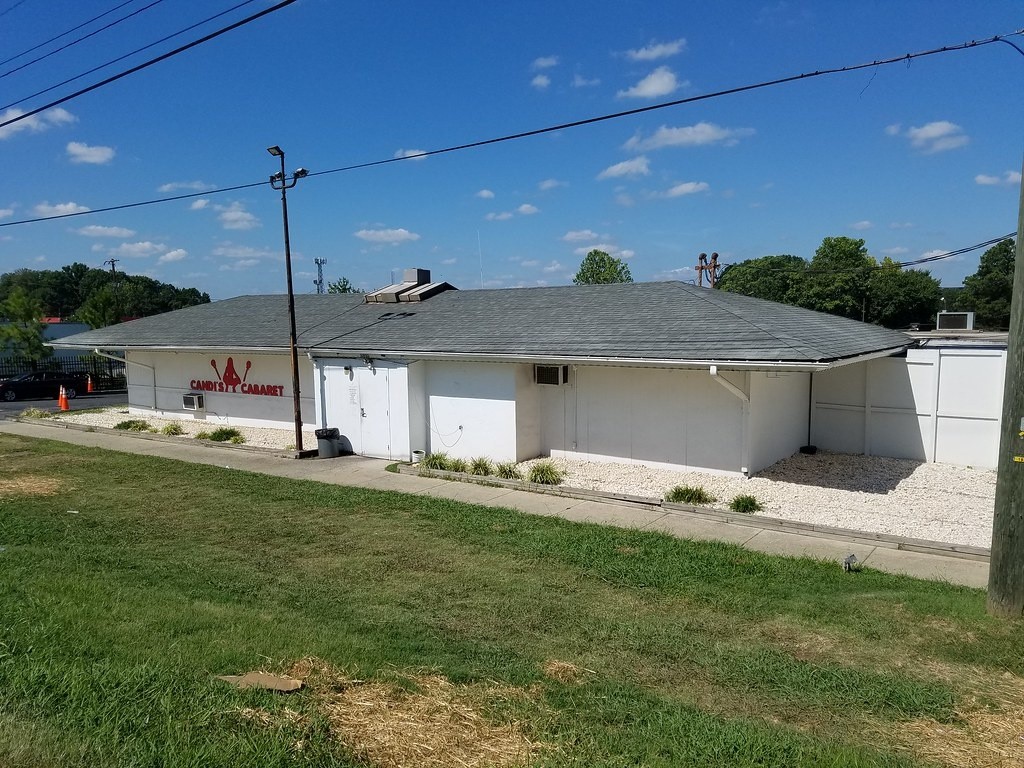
left=412, top=450, right=426, bottom=464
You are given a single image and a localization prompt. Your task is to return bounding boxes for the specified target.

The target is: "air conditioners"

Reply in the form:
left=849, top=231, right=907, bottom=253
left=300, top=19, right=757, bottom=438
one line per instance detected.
left=936, top=309, right=976, bottom=330
left=535, top=364, right=568, bottom=387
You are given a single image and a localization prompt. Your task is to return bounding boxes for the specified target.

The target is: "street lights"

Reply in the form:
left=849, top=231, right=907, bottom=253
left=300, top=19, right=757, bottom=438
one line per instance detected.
left=267, top=145, right=310, bottom=450
left=60, top=388, right=71, bottom=411
left=57, top=385, right=63, bottom=407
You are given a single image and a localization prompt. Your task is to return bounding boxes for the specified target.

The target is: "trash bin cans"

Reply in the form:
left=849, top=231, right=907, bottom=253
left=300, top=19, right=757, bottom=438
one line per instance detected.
left=315, top=428, right=340, bottom=459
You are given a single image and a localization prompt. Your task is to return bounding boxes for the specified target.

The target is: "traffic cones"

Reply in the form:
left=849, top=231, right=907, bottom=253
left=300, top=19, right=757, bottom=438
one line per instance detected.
left=87, top=377, right=94, bottom=393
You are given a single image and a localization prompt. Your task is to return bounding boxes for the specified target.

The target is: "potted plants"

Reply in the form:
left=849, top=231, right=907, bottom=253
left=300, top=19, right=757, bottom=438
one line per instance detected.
left=182, top=394, right=204, bottom=411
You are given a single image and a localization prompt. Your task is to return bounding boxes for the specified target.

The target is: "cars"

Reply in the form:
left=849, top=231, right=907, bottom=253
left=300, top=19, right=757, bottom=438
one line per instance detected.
left=0, top=371, right=88, bottom=402
